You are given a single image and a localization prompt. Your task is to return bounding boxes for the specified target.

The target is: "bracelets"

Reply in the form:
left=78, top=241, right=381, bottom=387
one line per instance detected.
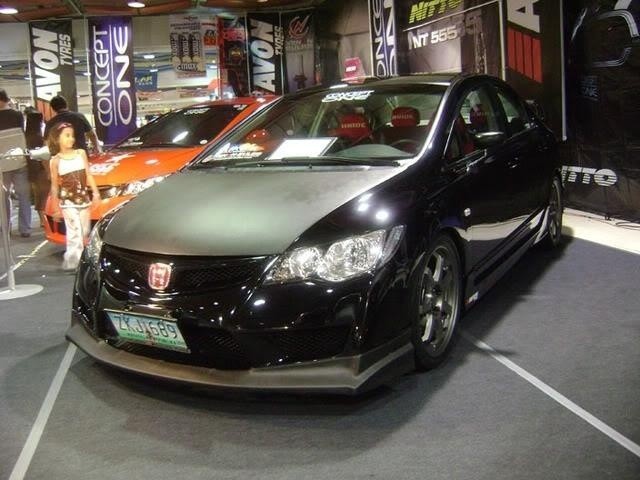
left=92, top=193, right=101, bottom=198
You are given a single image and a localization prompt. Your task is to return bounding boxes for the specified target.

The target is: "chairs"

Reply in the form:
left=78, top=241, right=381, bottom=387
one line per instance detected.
left=327, top=102, right=488, bottom=169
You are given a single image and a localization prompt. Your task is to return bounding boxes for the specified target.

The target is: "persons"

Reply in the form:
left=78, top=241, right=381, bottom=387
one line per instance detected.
left=46, top=120, right=101, bottom=272
left=1, top=89, right=31, bottom=238
left=42, top=94, right=98, bottom=182
left=22, top=105, right=46, bottom=140
left=23, top=109, right=50, bottom=229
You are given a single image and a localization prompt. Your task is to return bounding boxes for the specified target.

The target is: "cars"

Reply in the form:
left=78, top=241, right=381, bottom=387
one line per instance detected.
left=63, top=68, right=564, bottom=399
left=41, top=93, right=281, bottom=249
